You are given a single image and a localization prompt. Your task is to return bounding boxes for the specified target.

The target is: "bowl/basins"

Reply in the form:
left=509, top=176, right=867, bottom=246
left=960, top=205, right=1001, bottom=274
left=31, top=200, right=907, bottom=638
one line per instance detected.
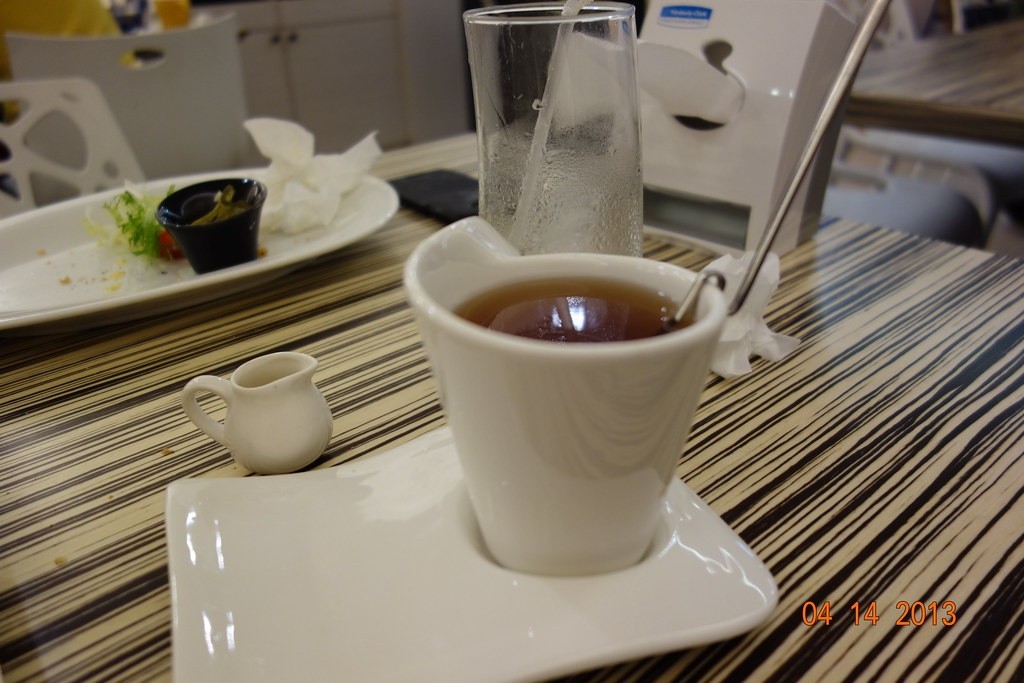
left=155, top=178, right=267, bottom=276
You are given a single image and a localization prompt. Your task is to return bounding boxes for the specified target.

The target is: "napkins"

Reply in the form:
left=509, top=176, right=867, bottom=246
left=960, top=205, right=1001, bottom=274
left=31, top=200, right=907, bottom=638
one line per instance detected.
left=237, top=118, right=383, bottom=234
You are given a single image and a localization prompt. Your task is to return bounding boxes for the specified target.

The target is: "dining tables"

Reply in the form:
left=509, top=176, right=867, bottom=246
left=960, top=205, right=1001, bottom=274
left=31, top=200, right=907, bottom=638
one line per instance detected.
left=0, top=134, right=1024, bottom=683
left=840, top=20, right=1024, bottom=143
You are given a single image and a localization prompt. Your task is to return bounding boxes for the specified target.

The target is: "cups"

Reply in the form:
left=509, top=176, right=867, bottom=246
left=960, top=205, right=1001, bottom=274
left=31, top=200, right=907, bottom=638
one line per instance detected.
left=462, top=3, right=642, bottom=258
left=402, top=218, right=729, bottom=575
left=7, top=10, right=245, bottom=206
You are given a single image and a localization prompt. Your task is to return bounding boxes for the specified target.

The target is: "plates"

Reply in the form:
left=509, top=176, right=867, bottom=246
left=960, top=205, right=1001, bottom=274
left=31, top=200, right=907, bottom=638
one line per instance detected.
left=167, top=428, right=777, bottom=683
left=0, top=166, right=398, bottom=330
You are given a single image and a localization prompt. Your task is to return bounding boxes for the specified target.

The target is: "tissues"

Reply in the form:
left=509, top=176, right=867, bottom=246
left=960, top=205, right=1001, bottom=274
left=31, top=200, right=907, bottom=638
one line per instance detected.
left=636, top=0, right=857, bottom=261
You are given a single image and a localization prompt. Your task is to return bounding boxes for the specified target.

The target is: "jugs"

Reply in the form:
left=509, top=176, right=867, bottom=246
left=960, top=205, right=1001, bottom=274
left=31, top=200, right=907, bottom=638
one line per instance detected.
left=185, top=353, right=333, bottom=473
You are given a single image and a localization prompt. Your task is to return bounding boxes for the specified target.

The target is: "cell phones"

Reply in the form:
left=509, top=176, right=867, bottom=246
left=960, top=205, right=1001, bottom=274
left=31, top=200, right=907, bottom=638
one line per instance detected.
left=388, top=167, right=479, bottom=224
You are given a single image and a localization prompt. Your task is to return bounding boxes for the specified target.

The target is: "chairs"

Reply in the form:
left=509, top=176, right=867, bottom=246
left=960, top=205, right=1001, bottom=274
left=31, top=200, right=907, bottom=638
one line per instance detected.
left=0, top=73, right=150, bottom=220
left=0, top=14, right=250, bottom=200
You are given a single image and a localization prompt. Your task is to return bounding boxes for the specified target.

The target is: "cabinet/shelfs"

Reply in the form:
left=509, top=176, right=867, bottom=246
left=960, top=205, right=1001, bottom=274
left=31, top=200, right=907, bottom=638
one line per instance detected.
left=229, top=0, right=420, bottom=158
